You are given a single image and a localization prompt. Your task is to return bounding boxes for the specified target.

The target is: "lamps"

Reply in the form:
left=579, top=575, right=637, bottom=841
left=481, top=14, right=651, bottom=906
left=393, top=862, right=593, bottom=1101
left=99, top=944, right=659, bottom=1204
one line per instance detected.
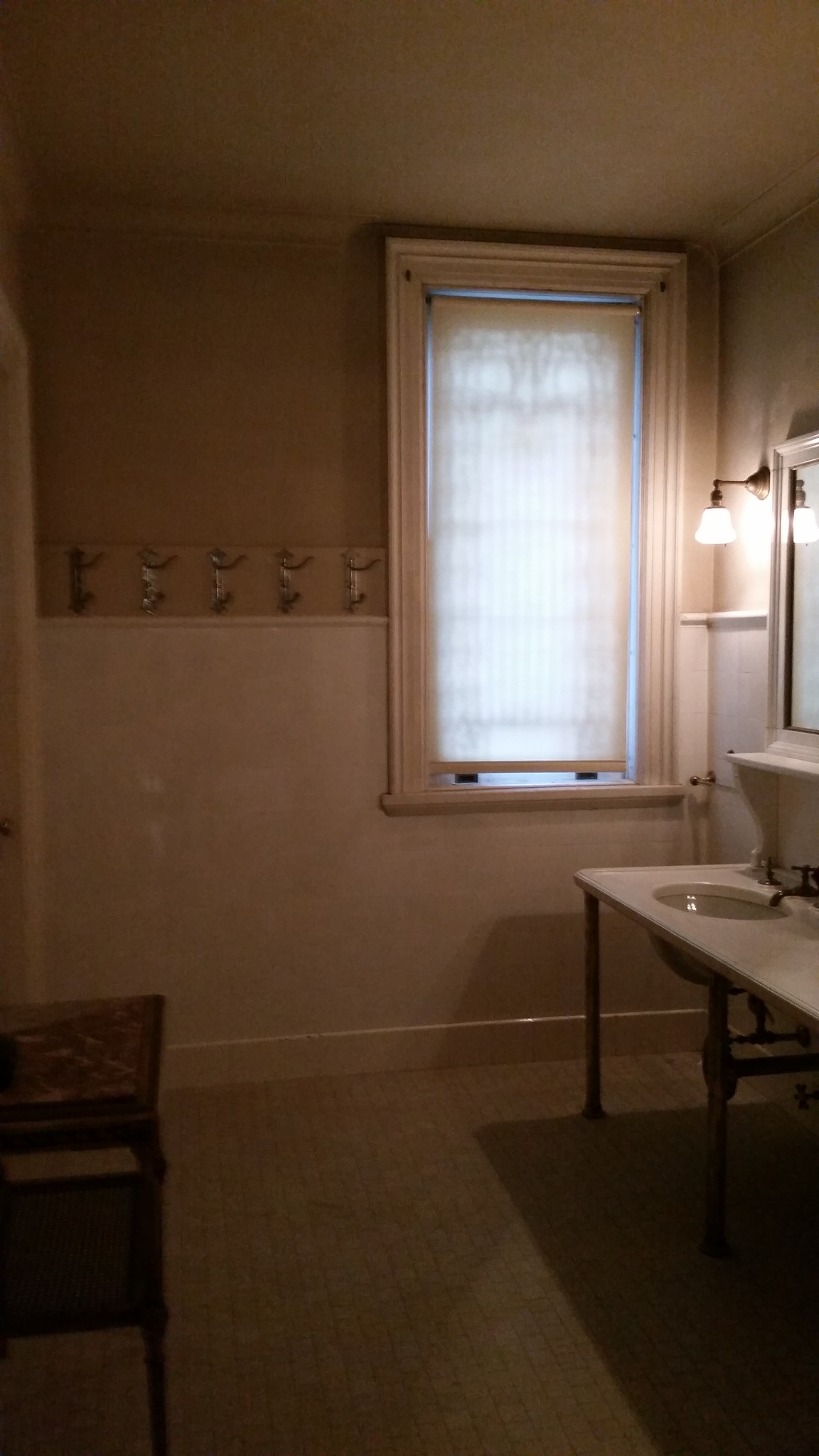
left=695, top=464, right=770, bottom=544
left=792, top=479, right=819, bottom=544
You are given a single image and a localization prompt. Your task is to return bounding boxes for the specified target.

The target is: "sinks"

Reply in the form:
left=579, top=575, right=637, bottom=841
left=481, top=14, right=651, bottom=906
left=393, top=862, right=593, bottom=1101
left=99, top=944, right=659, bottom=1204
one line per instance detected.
left=649, top=877, right=799, bottom=926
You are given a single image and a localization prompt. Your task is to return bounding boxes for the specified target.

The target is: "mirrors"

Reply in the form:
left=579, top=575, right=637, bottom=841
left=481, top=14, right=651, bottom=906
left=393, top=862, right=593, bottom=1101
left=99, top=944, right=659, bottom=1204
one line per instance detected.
left=762, top=429, right=819, bottom=764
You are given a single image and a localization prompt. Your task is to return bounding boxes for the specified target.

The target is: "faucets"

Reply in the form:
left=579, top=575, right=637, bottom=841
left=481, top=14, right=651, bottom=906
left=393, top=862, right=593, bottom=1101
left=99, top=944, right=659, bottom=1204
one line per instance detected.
left=766, top=862, right=819, bottom=908
